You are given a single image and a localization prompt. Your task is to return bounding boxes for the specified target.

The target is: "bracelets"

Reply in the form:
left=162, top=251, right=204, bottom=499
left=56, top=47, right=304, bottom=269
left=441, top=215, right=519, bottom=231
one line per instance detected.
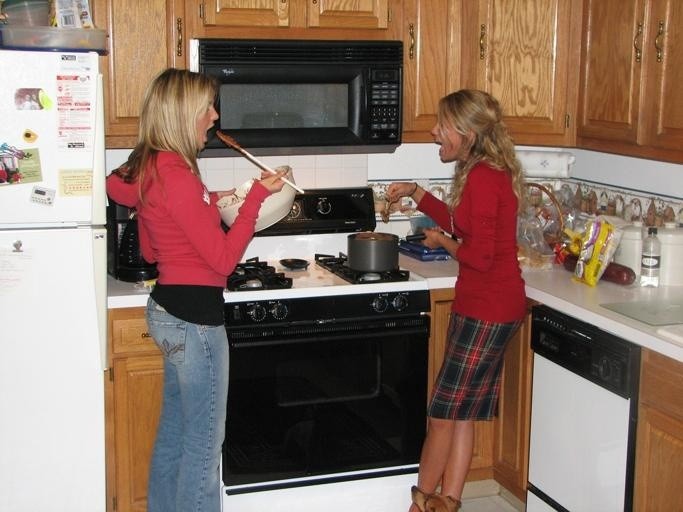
left=409, top=181, right=417, bottom=199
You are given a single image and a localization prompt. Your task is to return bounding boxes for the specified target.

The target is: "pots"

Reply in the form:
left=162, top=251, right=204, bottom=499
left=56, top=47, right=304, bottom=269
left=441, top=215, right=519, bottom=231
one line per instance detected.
left=345, top=232, right=399, bottom=272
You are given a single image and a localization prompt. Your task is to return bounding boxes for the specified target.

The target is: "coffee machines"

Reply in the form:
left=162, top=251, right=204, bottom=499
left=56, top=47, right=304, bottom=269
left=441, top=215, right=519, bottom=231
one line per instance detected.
left=107, top=190, right=158, bottom=284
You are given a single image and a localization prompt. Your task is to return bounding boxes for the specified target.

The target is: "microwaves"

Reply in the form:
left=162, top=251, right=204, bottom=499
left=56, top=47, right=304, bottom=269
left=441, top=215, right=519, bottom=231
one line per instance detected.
left=189, top=37, right=401, bottom=153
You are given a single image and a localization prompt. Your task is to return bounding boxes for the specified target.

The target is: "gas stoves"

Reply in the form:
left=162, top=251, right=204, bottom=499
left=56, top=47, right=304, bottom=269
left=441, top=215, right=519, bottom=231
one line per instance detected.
left=212, top=185, right=430, bottom=305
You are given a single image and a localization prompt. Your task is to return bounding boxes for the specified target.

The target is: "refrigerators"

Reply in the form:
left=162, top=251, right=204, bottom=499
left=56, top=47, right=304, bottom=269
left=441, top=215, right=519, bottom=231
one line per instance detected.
left=3, top=24, right=107, bottom=512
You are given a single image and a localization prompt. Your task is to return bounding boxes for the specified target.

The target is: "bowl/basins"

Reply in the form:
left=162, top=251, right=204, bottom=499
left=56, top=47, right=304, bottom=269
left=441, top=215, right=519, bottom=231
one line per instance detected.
left=3, top=2, right=51, bottom=26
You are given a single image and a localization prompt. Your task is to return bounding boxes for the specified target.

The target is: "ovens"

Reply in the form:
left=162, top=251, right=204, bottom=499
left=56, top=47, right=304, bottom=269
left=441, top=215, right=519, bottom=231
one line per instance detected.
left=219, top=290, right=431, bottom=480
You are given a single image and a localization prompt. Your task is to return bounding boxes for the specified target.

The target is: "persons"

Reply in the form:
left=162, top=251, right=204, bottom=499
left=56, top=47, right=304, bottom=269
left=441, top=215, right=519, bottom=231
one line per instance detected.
left=104, top=67, right=289, bottom=512
left=385, top=87, right=530, bottom=512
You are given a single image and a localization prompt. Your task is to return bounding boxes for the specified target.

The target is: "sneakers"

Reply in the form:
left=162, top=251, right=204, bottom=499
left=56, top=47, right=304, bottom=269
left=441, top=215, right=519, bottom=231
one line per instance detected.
left=425, top=495, right=462, bottom=512
left=409, top=485, right=436, bottom=512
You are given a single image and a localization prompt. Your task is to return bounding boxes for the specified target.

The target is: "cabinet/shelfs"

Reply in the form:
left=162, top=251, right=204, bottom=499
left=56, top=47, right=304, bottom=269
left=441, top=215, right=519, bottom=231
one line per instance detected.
left=576, top=0, right=682, bottom=165
left=465, top=0, right=576, bottom=149
left=187, top=0, right=394, bottom=36
left=89, top=0, right=193, bottom=152
left=104, top=307, right=164, bottom=511
left=427, top=288, right=495, bottom=483
left=393, top=0, right=458, bottom=144
left=493, top=292, right=532, bottom=500
left=631, top=342, right=682, bottom=512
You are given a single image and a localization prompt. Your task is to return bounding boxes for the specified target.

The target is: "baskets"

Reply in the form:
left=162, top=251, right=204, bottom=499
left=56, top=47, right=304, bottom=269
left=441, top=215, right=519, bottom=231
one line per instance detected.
left=516, top=182, right=566, bottom=267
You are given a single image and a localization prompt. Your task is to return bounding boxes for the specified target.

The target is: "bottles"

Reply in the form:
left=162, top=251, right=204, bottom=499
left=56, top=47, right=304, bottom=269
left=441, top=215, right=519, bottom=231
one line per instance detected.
left=640, top=228, right=661, bottom=287
